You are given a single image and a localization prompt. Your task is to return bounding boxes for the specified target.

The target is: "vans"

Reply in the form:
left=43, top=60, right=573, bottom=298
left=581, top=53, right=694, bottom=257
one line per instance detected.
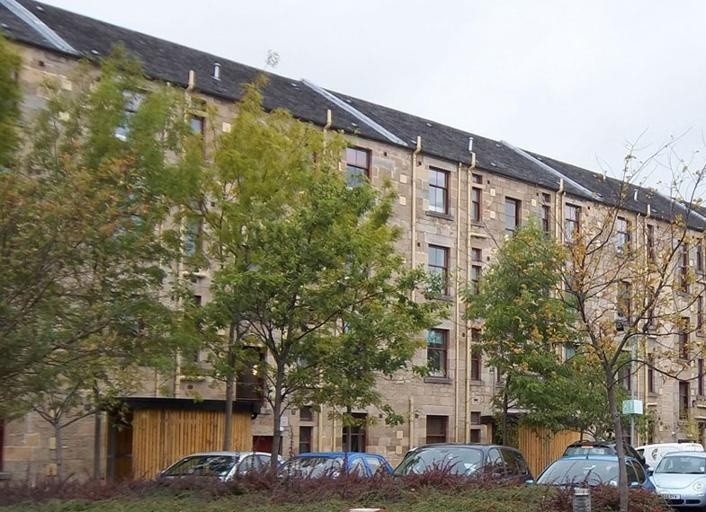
left=633, top=444, right=704, bottom=474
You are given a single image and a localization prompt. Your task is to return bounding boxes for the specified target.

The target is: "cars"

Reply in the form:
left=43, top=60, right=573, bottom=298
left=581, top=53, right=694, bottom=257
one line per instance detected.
left=277, top=451, right=394, bottom=483
left=155, top=452, right=284, bottom=488
left=647, top=452, right=705, bottom=509
left=524, top=455, right=655, bottom=497
left=390, top=443, right=533, bottom=487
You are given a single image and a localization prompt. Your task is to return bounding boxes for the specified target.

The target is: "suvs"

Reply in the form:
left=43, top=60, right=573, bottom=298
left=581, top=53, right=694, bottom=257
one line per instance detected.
left=562, top=439, right=649, bottom=473
